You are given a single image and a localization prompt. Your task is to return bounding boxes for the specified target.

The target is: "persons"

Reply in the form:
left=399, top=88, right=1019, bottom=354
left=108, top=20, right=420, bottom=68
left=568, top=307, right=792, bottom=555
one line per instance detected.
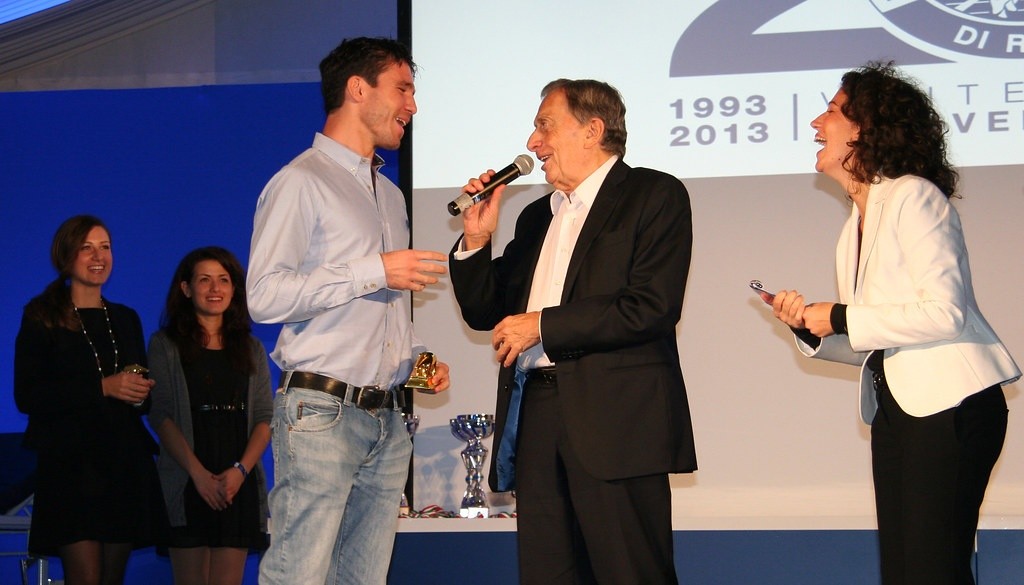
left=245, top=36, right=450, bottom=585
left=12, top=215, right=172, bottom=585
left=146, top=247, right=273, bottom=585
left=760, top=56, right=1023, bottom=585
left=449, top=79, right=699, bottom=585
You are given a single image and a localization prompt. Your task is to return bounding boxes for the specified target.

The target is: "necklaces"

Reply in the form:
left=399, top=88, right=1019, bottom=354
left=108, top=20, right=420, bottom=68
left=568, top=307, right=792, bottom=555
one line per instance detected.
left=70, top=298, right=119, bottom=378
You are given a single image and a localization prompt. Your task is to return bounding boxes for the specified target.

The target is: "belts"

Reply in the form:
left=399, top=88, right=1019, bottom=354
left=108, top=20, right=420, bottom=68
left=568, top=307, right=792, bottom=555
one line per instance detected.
left=528, top=368, right=558, bottom=387
left=280, top=371, right=407, bottom=409
left=872, top=371, right=886, bottom=391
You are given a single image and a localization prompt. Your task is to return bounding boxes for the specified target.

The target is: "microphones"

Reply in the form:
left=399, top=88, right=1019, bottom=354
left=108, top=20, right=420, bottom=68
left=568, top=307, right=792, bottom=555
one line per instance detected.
left=449, top=154, right=534, bottom=216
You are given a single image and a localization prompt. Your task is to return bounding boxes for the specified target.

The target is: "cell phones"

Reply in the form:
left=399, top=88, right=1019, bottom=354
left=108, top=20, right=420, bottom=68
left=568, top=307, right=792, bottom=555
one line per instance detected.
left=749, top=280, right=778, bottom=297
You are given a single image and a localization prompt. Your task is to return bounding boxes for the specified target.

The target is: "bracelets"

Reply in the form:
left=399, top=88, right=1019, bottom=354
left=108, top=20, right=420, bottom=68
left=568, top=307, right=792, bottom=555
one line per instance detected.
left=234, top=462, right=248, bottom=478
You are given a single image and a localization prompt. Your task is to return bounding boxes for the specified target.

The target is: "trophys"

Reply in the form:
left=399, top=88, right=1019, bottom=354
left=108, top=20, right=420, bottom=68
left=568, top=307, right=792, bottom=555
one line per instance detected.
left=449, top=413, right=496, bottom=518
left=399, top=414, right=420, bottom=517
left=404, top=352, right=439, bottom=390
